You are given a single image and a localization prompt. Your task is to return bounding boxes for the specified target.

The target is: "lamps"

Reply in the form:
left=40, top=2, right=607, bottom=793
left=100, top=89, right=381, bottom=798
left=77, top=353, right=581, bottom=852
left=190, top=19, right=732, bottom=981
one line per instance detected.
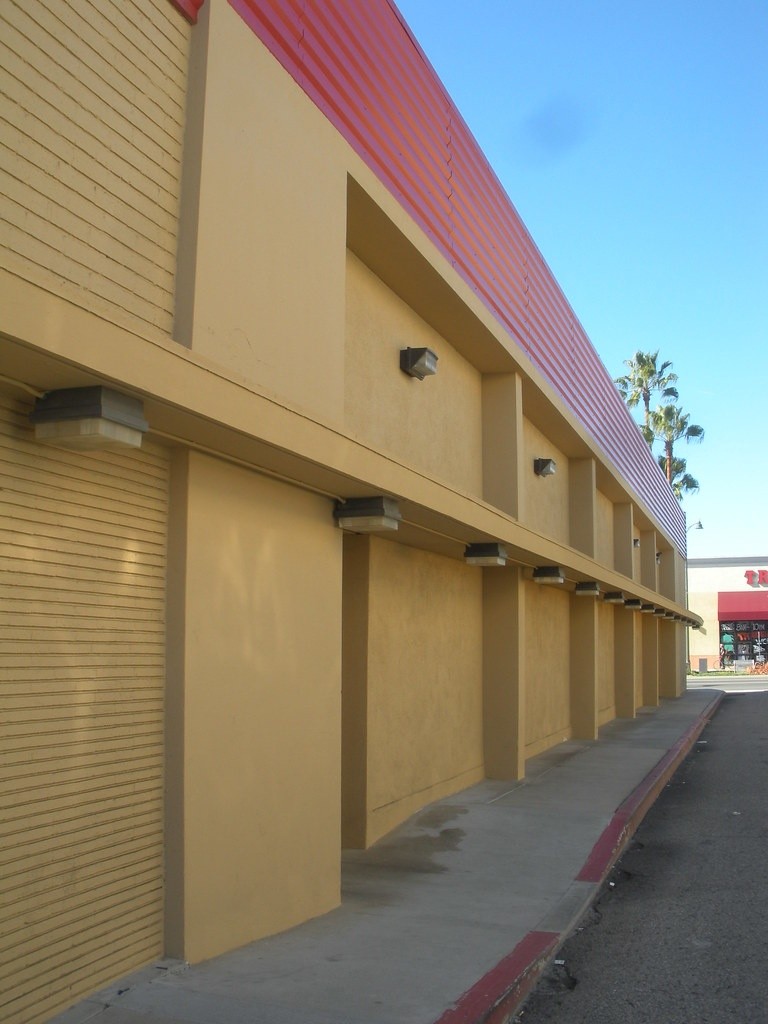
left=400, top=346, right=439, bottom=381
left=534, top=458, right=557, bottom=478
left=332, top=494, right=701, bottom=631
left=29, top=385, right=147, bottom=454
